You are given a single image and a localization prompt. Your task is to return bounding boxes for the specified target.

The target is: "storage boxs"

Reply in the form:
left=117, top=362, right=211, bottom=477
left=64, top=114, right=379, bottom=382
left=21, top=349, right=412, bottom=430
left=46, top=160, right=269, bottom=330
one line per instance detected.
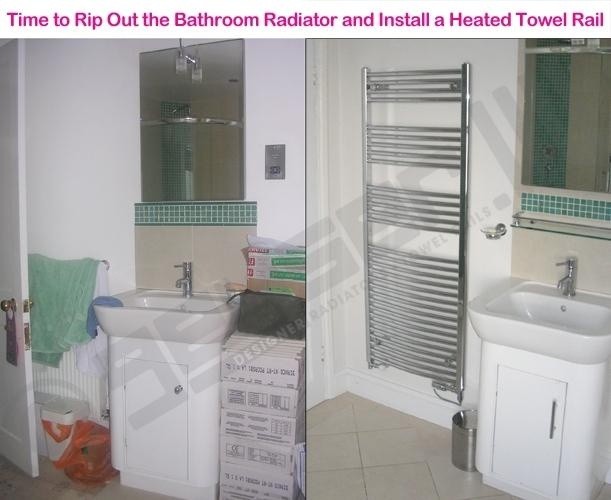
left=242, top=245, right=305, bottom=306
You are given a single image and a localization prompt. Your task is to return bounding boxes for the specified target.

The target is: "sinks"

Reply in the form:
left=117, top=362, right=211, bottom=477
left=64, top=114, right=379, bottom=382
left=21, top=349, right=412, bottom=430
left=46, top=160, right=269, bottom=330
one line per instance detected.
left=467, top=276, right=611, bottom=364
left=93, top=287, right=239, bottom=342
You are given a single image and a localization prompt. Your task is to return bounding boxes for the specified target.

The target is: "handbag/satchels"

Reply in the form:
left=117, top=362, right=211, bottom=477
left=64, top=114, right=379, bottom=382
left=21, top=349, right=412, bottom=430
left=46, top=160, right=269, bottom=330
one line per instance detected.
left=238, top=288, right=305, bottom=340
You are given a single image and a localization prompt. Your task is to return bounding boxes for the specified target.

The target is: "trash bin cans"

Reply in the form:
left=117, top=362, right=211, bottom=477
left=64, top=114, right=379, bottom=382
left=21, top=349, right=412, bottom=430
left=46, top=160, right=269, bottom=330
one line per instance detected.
left=451, top=409, right=477, bottom=472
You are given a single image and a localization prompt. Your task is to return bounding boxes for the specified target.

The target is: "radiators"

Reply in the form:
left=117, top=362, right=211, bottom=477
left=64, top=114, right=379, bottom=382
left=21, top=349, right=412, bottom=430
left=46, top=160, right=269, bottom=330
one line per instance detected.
left=31, top=326, right=107, bottom=424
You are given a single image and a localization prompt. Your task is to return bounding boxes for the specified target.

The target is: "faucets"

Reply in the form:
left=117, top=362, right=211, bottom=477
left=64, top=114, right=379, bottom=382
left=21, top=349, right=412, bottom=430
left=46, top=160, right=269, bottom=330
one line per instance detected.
left=555, top=257, right=577, bottom=297
left=173, top=262, right=193, bottom=297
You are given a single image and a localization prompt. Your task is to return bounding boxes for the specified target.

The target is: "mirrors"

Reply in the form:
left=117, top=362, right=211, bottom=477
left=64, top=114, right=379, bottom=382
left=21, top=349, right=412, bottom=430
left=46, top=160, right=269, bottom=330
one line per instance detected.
left=138, top=38, right=246, bottom=203
left=517, top=39, right=611, bottom=195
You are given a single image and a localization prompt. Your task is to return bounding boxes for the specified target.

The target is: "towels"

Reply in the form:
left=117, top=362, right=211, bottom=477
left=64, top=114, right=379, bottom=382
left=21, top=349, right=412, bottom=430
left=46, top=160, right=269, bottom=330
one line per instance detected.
left=84, top=294, right=123, bottom=340
left=26, top=252, right=98, bottom=367
left=74, top=261, right=113, bottom=379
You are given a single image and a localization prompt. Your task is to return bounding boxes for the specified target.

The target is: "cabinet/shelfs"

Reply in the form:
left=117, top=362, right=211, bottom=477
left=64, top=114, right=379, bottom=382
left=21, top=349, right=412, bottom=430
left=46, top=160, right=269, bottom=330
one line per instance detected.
left=475, top=341, right=610, bottom=500
left=108, top=336, right=222, bottom=499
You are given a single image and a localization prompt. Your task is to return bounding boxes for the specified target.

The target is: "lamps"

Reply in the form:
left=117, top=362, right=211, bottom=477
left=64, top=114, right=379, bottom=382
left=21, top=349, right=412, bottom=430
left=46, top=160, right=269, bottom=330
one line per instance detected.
left=175, top=40, right=206, bottom=86
